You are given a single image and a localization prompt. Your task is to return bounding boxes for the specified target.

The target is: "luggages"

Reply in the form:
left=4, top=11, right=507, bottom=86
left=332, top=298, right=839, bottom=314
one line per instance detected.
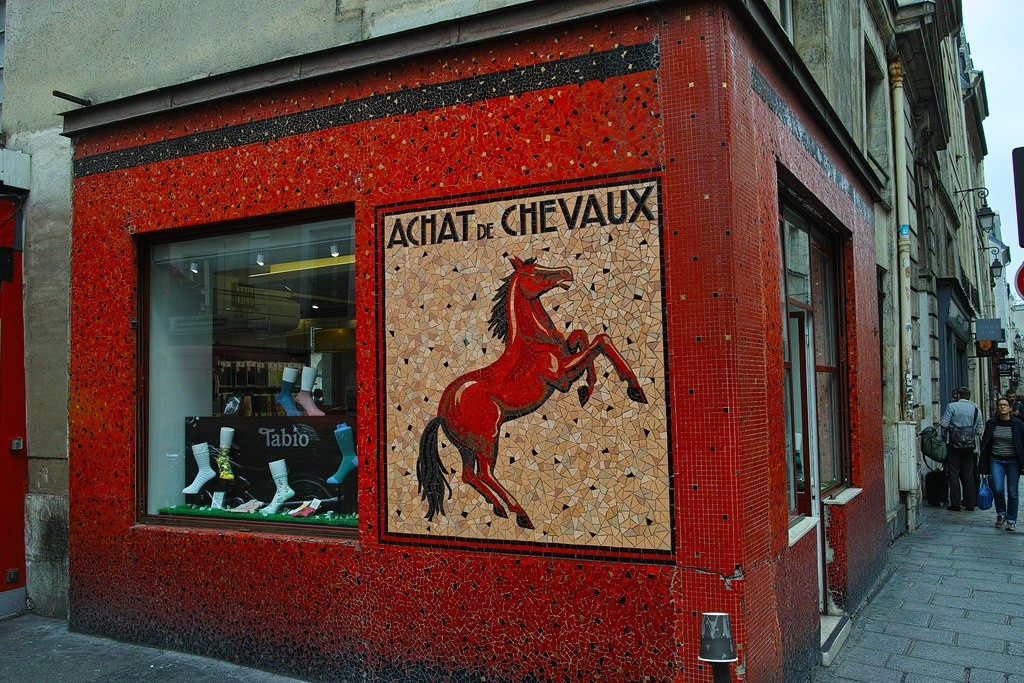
left=925, top=468, right=949, bottom=506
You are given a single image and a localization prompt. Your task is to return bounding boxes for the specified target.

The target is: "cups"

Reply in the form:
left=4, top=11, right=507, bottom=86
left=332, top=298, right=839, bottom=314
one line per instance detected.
left=698, top=612, right=739, bottom=662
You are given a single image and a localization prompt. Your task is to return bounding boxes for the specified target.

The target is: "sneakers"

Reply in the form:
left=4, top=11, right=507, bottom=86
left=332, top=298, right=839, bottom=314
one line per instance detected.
left=995, top=518, right=1005, bottom=528
left=1006, top=523, right=1016, bottom=531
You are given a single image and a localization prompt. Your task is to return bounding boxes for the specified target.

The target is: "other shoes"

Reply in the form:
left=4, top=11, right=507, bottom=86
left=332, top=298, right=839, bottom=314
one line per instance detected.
left=965, top=506, right=974, bottom=511
left=946, top=506, right=960, bottom=511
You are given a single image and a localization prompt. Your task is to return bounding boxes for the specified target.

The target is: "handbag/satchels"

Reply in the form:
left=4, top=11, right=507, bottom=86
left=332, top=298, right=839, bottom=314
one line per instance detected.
left=977, top=474, right=994, bottom=510
left=920, top=422, right=947, bottom=463
left=949, top=408, right=978, bottom=450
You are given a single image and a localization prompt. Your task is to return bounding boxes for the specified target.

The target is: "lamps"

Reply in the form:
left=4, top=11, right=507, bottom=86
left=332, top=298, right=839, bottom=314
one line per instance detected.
left=191, top=260, right=199, bottom=274
left=257, top=251, right=264, bottom=266
left=976, top=246, right=1004, bottom=278
left=330, top=242, right=339, bottom=257
left=952, top=187, right=996, bottom=233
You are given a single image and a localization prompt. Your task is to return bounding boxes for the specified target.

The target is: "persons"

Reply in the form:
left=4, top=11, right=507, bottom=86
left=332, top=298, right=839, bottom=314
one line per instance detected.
left=978, top=398, right=1024, bottom=531
left=326, top=426, right=358, bottom=484
left=940, top=387, right=982, bottom=511
left=182, top=443, right=216, bottom=494
left=294, top=366, right=326, bottom=416
left=216, top=428, right=234, bottom=479
left=995, top=389, right=1024, bottom=422
left=275, top=366, right=302, bottom=416
left=259, top=460, right=295, bottom=514
